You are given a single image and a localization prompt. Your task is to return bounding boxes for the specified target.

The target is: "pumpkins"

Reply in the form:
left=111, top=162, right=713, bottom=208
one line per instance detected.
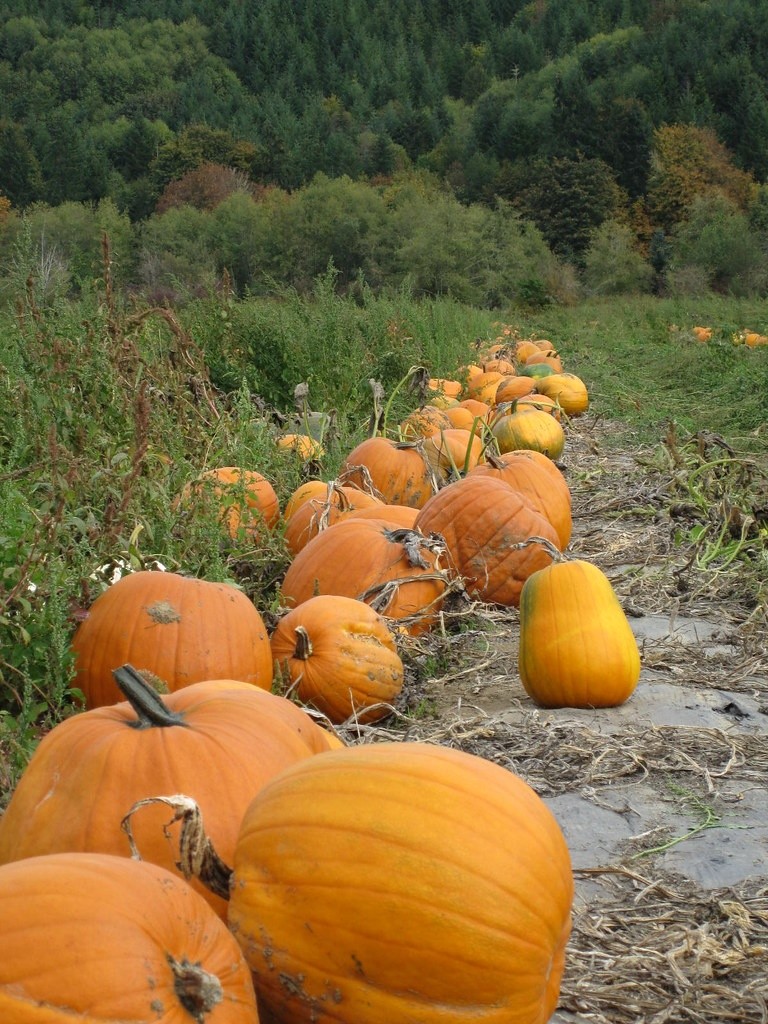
left=171, top=322, right=768, bottom=726
left=0, top=666, right=573, bottom=1024
left=67, top=570, right=273, bottom=712
